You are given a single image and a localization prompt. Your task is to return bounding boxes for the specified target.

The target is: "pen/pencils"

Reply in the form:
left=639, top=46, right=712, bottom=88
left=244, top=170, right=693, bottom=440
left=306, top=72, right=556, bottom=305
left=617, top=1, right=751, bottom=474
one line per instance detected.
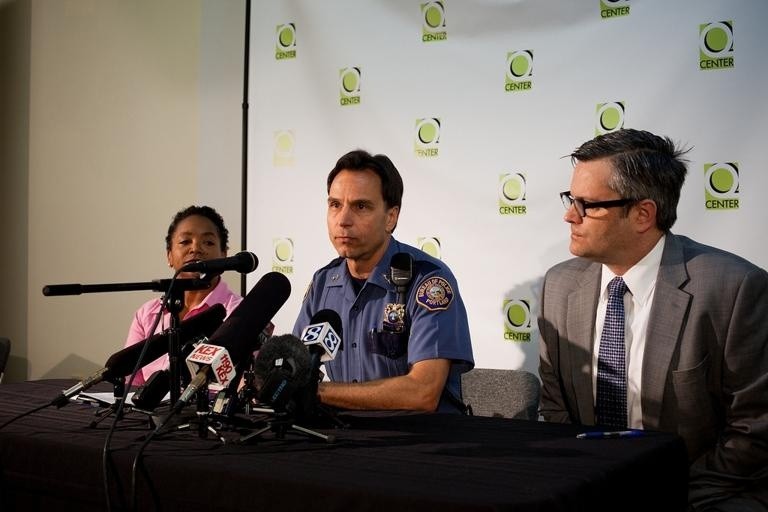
left=576, top=429, right=648, bottom=439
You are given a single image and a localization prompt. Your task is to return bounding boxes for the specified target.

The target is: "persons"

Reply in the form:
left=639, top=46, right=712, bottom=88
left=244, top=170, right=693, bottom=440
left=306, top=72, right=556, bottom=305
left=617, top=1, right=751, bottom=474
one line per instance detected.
left=293, top=151, right=475, bottom=418
left=541, top=129, right=768, bottom=512
left=117, top=207, right=273, bottom=395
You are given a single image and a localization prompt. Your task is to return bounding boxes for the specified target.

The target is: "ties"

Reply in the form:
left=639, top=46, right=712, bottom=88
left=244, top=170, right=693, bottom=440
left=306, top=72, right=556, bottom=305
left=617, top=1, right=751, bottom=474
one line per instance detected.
left=596, top=277, right=628, bottom=428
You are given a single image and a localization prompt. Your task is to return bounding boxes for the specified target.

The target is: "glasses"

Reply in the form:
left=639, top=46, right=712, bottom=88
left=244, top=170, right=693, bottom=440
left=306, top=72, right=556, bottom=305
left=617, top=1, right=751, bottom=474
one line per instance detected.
left=560, top=191, right=633, bottom=217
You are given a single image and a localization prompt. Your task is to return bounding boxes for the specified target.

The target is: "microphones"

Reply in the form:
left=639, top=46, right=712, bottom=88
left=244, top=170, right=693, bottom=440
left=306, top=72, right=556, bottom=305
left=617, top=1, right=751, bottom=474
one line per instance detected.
left=299, top=310, right=342, bottom=375
left=65, top=303, right=227, bottom=399
left=212, top=320, right=275, bottom=415
left=173, top=272, right=291, bottom=413
left=182, top=250, right=258, bottom=274
left=253, top=334, right=311, bottom=417
